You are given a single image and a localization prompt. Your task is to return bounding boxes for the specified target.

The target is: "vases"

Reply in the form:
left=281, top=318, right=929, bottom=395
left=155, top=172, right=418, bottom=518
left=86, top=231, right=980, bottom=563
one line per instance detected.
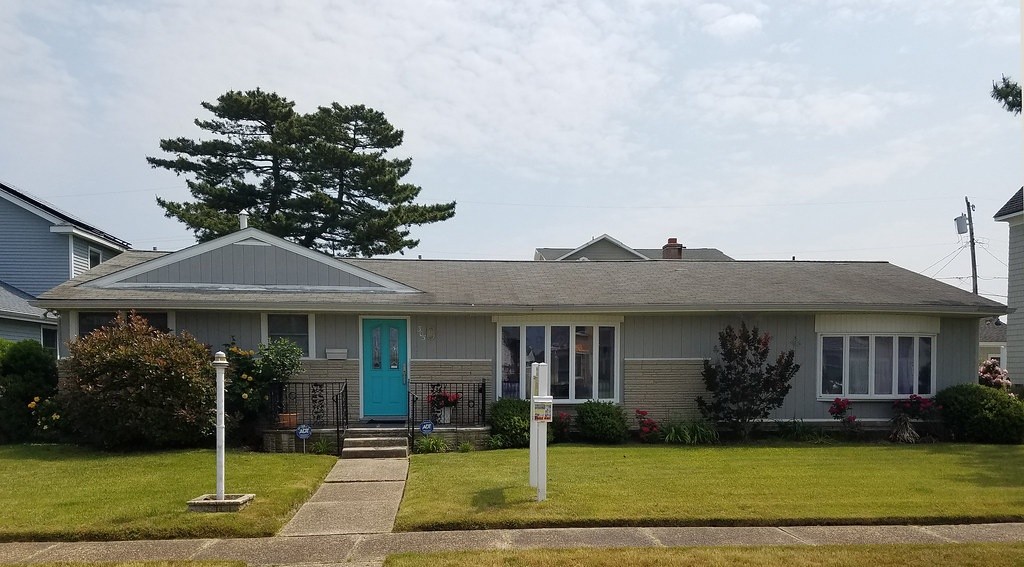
left=433, top=407, right=453, bottom=424
left=279, top=412, right=302, bottom=429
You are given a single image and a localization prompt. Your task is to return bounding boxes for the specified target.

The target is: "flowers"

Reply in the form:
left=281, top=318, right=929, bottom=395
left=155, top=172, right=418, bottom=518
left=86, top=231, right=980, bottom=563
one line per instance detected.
left=636, top=408, right=658, bottom=433
left=426, top=391, right=460, bottom=408
left=828, top=397, right=861, bottom=430
left=892, top=395, right=935, bottom=415
